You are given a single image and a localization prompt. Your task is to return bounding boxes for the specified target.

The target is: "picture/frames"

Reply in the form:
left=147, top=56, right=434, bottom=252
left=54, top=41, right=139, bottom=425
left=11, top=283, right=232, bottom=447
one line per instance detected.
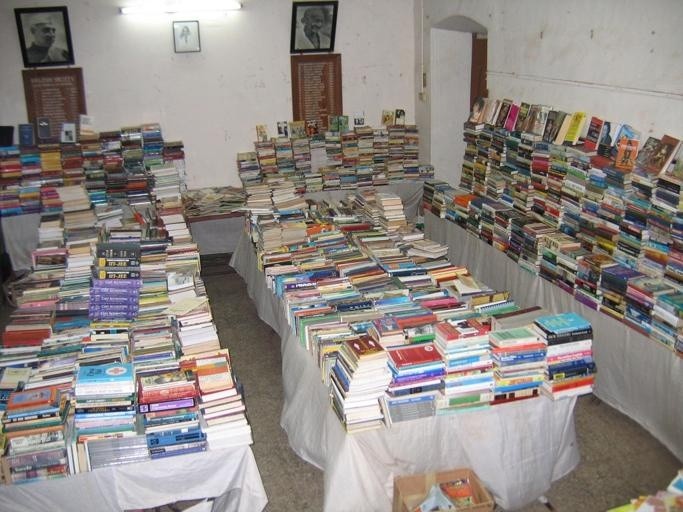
left=290, top=1, right=339, bottom=52
left=172, top=21, right=201, bottom=54
left=13, top=6, right=75, bottom=67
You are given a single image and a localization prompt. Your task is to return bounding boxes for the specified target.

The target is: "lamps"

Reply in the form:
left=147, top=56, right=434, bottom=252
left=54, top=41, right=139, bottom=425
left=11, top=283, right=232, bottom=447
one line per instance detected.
left=118, top=1, right=244, bottom=15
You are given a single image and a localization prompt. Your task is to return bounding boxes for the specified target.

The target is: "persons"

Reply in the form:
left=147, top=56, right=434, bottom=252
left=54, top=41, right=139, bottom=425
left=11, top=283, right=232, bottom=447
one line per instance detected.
left=25, top=11, right=71, bottom=63
left=295, top=6, right=331, bottom=50
left=177, top=25, right=197, bottom=50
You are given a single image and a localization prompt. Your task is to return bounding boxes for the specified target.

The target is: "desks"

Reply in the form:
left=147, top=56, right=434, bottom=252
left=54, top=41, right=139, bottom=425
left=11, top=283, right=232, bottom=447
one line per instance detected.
left=2, top=212, right=41, bottom=271
left=0, top=445, right=268, bottom=512
left=228, top=227, right=579, bottom=512
left=188, top=211, right=246, bottom=255
left=303, top=177, right=434, bottom=224
left=423, top=208, right=683, bottom=465
